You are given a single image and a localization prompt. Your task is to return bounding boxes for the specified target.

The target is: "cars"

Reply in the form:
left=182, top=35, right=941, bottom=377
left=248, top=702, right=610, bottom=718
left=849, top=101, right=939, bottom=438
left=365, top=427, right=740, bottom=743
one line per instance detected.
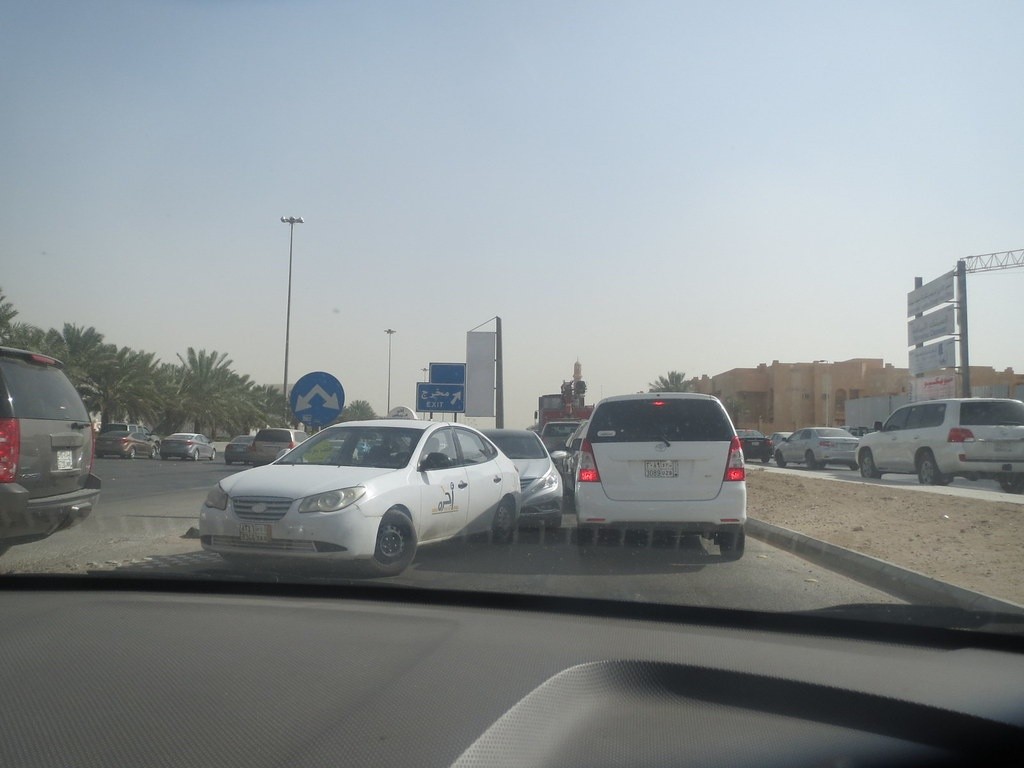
left=159, top=432, right=217, bottom=461
left=310, top=438, right=382, bottom=466
left=96, top=431, right=159, bottom=460
left=538, top=420, right=588, bottom=496
left=198, top=420, right=522, bottom=577
left=771, top=431, right=794, bottom=447
left=735, top=429, right=773, bottom=463
left=224, top=436, right=254, bottom=465
left=773, top=427, right=860, bottom=471
left=474, top=427, right=563, bottom=534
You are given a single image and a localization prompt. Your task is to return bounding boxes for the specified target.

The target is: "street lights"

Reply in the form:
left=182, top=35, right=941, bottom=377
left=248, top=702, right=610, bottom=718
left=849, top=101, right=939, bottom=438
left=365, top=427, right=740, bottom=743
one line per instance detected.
left=280, top=214, right=305, bottom=429
left=384, top=328, right=397, bottom=413
left=421, top=368, right=428, bottom=420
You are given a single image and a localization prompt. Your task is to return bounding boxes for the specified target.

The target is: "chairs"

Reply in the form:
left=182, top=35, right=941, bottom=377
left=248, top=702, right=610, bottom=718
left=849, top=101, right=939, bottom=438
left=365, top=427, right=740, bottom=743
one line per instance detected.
left=418, top=452, right=451, bottom=471
left=361, top=447, right=393, bottom=468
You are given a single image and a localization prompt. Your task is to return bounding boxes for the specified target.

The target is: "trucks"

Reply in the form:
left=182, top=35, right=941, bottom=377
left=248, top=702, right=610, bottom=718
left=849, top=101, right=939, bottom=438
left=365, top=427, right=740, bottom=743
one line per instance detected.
left=533, top=393, right=596, bottom=437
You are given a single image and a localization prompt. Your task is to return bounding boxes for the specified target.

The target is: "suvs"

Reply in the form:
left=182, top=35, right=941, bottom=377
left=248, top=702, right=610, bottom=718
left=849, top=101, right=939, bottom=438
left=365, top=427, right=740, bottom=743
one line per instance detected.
left=248, top=429, right=306, bottom=467
left=1, top=346, right=102, bottom=557
left=575, top=392, right=747, bottom=560
left=98, top=422, right=161, bottom=448
left=855, top=397, right=1024, bottom=495
